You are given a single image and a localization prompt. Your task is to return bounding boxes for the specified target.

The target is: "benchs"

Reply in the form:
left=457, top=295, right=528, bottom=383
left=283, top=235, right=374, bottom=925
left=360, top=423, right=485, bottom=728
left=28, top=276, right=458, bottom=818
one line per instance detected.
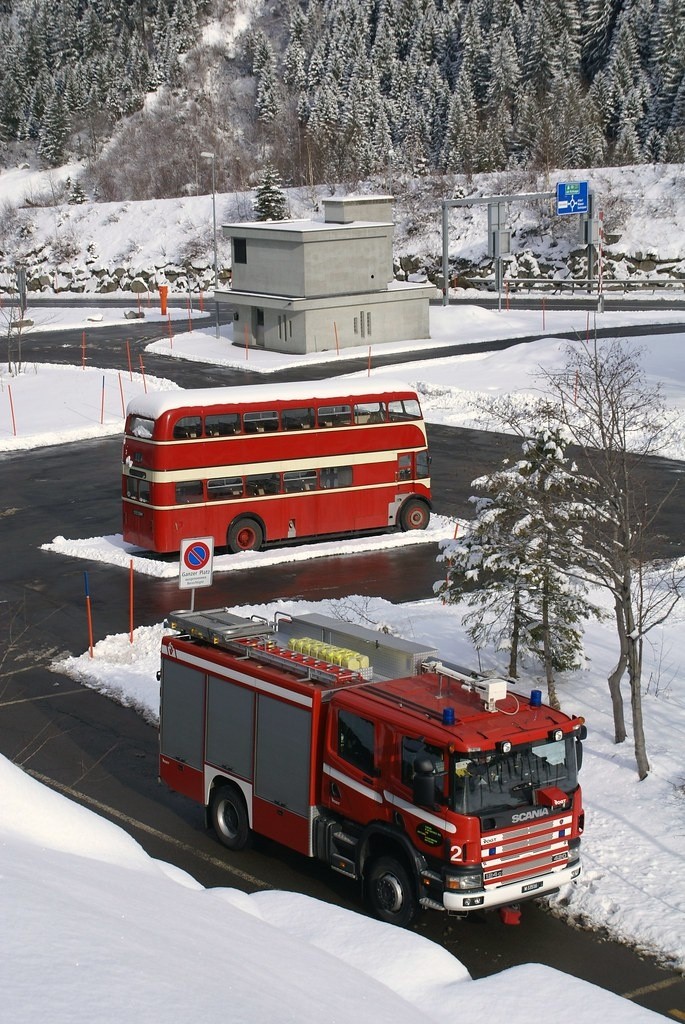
left=219, top=422, right=241, bottom=434
left=192, top=427, right=219, bottom=437
left=306, top=415, right=333, bottom=427
left=285, top=416, right=310, bottom=429
left=284, top=475, right=311, bottom=491
left=233, top=421, right=264, bottom=432
left=175, top=426, right=196, bottom=438
left=265, top=478, right=287, bottom=493
left=247, top=482, right=264, bottom=495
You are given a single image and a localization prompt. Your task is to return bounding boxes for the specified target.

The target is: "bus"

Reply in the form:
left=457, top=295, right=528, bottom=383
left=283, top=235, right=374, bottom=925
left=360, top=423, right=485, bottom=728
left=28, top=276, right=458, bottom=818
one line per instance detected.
left=123, top=378, right=434, bottom=554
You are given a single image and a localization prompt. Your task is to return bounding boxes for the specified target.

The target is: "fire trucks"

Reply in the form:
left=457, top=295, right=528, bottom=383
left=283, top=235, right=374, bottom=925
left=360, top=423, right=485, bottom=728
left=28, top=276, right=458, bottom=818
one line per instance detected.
left=157, top=602, right=588, bottom=929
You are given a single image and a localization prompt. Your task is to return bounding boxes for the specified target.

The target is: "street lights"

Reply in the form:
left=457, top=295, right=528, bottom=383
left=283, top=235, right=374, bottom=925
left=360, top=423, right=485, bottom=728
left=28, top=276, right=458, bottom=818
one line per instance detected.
left=201, top=151, right=220, bottom=339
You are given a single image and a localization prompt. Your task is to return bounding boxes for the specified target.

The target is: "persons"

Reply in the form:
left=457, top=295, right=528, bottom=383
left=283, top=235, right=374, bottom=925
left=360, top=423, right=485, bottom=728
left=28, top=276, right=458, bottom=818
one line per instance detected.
left=467, top=757, right=499, bottom=791
